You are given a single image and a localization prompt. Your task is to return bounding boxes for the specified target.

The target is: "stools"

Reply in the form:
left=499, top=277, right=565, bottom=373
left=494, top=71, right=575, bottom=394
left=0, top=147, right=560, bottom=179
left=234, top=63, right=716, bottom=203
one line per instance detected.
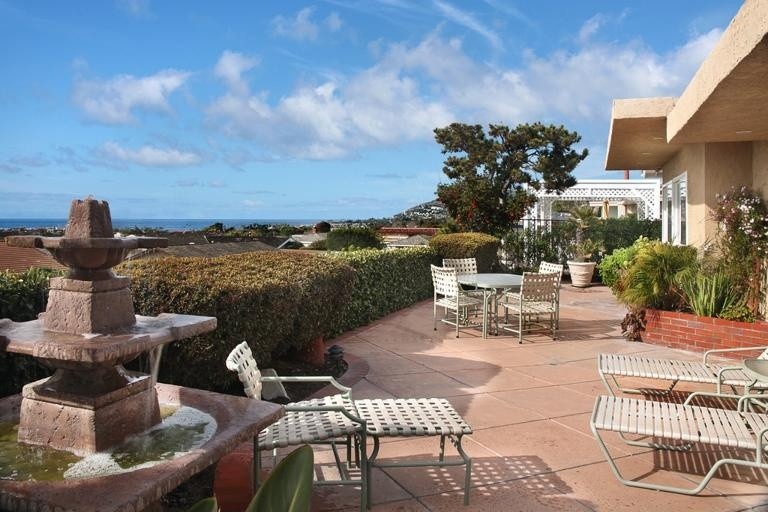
left=355, top=397, right=473, bottom=508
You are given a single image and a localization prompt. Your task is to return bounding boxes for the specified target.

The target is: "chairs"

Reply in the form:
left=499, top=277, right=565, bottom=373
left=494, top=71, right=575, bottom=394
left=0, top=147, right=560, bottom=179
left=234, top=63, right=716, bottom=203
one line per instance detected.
left=593, top=337, right=768, bottom=410
left=590, top=392, right=768, bottom=495
left=225, top=340, right=368, bottom=511
left=430, top=258, right=563, bottom=343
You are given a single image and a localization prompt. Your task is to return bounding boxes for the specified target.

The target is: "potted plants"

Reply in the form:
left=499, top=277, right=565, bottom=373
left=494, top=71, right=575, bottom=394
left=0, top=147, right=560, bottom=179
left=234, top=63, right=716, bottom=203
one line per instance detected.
left=566, top=239, right=603, bottom=288
